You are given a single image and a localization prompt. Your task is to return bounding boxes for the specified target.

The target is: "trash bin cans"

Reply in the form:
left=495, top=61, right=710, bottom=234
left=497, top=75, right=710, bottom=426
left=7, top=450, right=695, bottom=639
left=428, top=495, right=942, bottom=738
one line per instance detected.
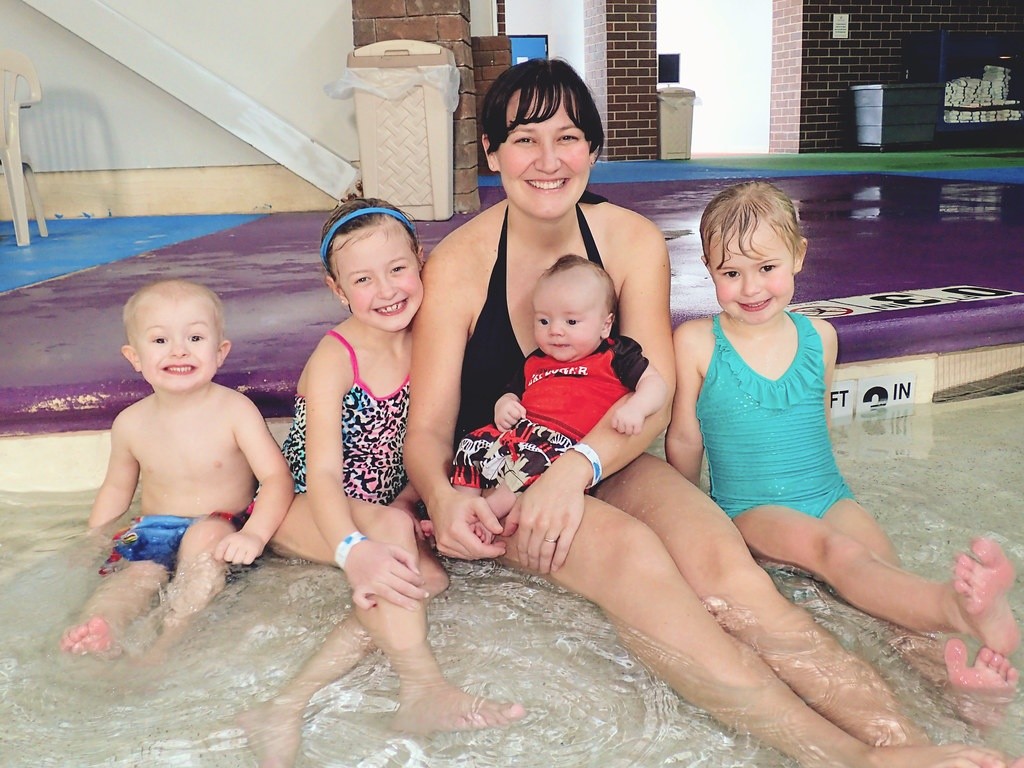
left=657, top=87, right=695, bottom=160
left=345, top=38, right=457, bottom=219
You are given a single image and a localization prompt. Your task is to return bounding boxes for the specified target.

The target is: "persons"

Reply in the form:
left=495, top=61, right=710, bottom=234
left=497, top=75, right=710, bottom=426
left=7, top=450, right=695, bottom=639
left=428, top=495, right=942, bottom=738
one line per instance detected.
left=664, top=179, right=1019, bottom=688
left=256, top=200, right=449, bottom=649
left=402, top=61, right=809, bottom=676
left=420, top=256, right=664, bottom=547
left=57, top=282, right=294, bottom=652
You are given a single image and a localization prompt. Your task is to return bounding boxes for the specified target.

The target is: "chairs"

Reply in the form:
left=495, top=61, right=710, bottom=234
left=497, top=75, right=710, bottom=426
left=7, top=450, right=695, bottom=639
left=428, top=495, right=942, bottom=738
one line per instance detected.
left=0, top=46, right=49, bottom=247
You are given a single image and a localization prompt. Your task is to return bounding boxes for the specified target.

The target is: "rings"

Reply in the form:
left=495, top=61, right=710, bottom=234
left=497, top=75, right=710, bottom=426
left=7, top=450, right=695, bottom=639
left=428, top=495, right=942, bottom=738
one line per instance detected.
left=543, top=538, right=557, bottom=543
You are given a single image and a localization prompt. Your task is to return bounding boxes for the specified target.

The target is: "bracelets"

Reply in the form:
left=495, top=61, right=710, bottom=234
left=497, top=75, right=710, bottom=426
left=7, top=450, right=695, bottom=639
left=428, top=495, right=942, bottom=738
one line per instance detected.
left=335, top=531, right=367, bottom=569
left=565, top=443, right=602, bottom=489
